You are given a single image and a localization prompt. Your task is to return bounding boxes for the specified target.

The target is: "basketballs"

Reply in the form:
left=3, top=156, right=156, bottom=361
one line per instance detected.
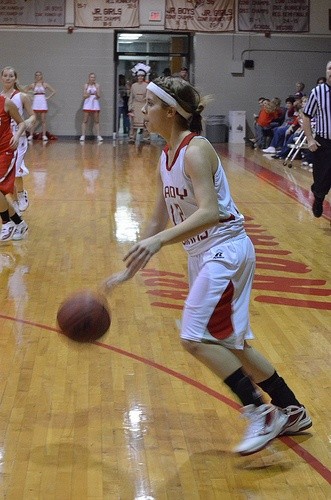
left=57, top=292, right=111, bottom=342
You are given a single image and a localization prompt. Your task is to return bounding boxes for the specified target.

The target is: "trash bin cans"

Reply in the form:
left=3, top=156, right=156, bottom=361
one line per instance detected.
left=228, top=110, right=246, bottom=144
left=206, top=114, right=226, bottom=143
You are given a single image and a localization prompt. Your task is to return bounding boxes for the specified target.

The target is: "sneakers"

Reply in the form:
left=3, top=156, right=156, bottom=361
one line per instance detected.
left=12, top=190, right=29, bottom=217
left=1, top=221, right=17, bottom=242
left=303, top=162, right=313, bottom=168
left=97, top=136, right=103, bottom=141
left=262, top=146, right=281, bottom=153
left=232, top=403, right=290, bottom=456
left=277, top=405, right=313, bottom=436
left=42, top=136, right=48, bottom=140
left=79, top=135, right=85, bottom=140
left=27, top=136, right=33, bottom=140
left=12, top=220, right=29, bottom=240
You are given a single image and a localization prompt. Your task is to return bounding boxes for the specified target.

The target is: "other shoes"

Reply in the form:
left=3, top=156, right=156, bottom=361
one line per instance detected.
left=270, top=156, right=281, bottom=159
left=244, top=137, right=255, bottom=149
left=124, top=129, right=151, bottom=143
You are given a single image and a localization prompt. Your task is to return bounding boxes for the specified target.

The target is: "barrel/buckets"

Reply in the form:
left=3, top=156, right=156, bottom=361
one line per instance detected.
left=205, top=115, right=226, bottom=142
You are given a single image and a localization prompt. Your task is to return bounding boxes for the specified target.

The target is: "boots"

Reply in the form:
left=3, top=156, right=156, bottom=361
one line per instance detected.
left=312, top=195, right=325, bottom=217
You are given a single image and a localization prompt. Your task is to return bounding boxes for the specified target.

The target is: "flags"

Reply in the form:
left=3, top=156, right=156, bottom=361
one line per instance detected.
left=73, top=0, right=140, bottom=28
left=238, top=0, right=311, bottom=32
left=163, top=0, right=236, bottom=33
left=0, top=0, right=67, bottom=27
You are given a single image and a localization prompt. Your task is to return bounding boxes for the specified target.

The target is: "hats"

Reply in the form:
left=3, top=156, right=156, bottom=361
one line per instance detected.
left=137, top=71, right=145, bottom=76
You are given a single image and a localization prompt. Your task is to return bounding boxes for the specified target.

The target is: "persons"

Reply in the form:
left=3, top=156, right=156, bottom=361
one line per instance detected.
left=303, top=60, right=331, bottom=218
left=102, top=74, right=313, bottom=456
left=0, top=66, right=36, bottom=218
left=0, top=95, right=30, bottom=242
left=316, top=77, right=326, bottom=85
left=243, top=80, right=313, bottom=169
left=174, top=67, right=188, bottom=81
left=117, top=75, right=130, bottom=134
left=127, top=71, right=152, bottom=145
left=78, top=73, right=104, bottom=142
left=24, top=71, right=57, bottom=142
